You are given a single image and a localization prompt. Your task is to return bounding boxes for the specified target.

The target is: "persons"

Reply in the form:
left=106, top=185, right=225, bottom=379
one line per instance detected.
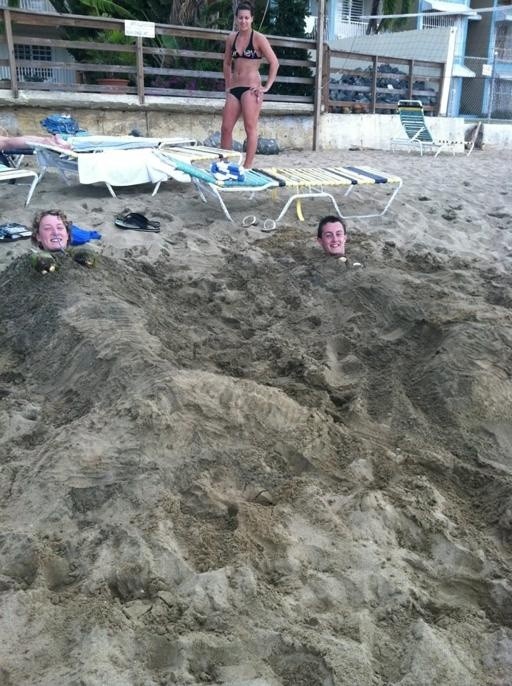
left=221, top=2, right=280, bottom=169
left=31, top=209, right=71, bottom=252
left=317, top=215, right=348, bottom=256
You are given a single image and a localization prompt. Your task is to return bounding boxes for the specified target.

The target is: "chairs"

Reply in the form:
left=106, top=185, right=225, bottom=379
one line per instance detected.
left=398, top=100, right=475, bottom=158
left=23, top=138, right=248, bottom=206
left=151, top=149, right=405, bottom=229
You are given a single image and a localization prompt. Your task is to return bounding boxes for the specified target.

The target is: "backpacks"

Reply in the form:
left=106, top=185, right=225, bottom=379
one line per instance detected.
left=39, top=114, right=80, bottom=135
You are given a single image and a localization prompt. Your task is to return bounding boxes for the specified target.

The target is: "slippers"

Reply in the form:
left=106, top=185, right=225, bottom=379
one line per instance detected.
left=114, top=217, right=160, bottom=233
left=115, top=212, right=161, bottom=227
left=242, top=215, right=259, bottom=228
left=261, top=219, right=282, bottom=232
left=0, top=230, right=33, bottom=242
left=1, top=223, right=28, bottom=232
